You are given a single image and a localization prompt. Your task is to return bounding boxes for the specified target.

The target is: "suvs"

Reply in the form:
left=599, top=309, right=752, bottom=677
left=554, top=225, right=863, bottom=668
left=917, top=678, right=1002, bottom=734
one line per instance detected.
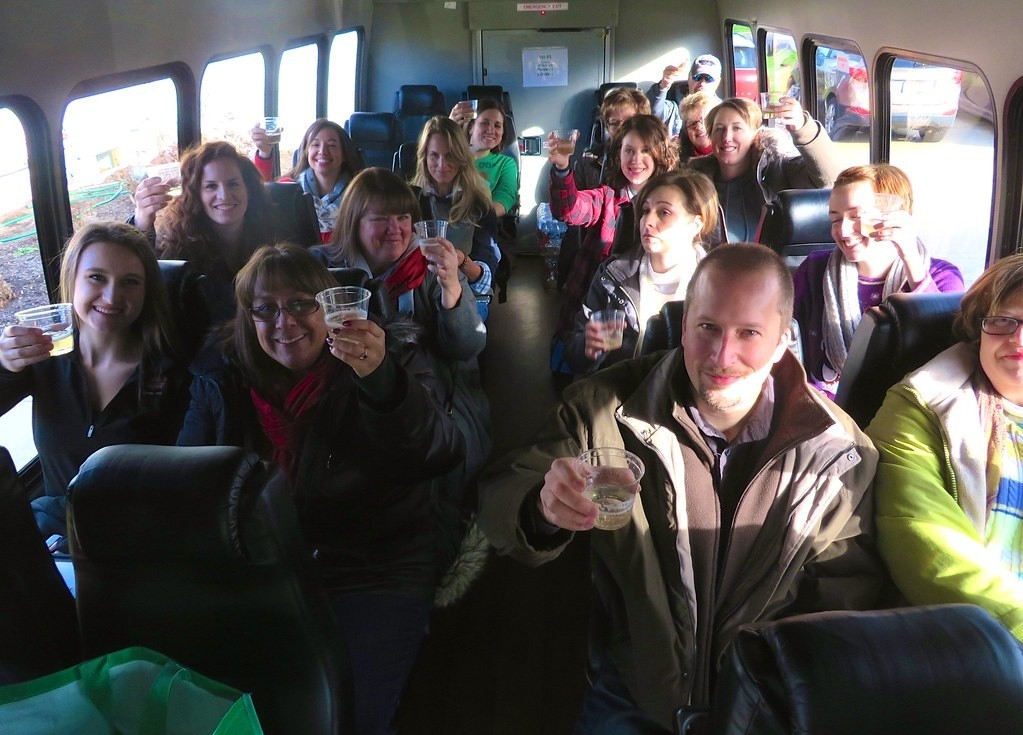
left=784, top=45, right=964, bottom=144
left=732, top=31, right=770, bottom=108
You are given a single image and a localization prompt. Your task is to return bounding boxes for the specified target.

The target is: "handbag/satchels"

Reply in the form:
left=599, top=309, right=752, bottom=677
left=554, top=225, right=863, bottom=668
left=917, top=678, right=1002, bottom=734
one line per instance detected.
left=0, top=646, right=264, bottom=735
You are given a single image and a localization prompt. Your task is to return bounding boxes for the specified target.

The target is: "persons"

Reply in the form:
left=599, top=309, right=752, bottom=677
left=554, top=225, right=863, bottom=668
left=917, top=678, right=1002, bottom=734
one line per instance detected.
left=863, top=254, right=1023, bottom=654
left=568, top=169, right=720, bottom=376
left=482, top=243, right=879, bottom=735
left=125, top=140, right=299, bottom=273
left=548, top=53, right=724, bottom=323
left=403, top=115, right=502, bottom=323
left=305, top=167, right=487, bottom=356
left=0, top=222, right=196, bottom=603
left=249, top=118, right=364, bottom=245
left=688, top=96, right=846, bottom=253
left=174, top=242, right=494, bottom=735
left=447, top=96, right=518, bottom=218
left=792, top=163, right=965, bottom=402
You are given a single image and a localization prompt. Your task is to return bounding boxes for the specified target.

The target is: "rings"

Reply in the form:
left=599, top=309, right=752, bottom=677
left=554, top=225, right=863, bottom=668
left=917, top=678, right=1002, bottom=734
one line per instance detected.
left=359, top=348, right=367, bottom=359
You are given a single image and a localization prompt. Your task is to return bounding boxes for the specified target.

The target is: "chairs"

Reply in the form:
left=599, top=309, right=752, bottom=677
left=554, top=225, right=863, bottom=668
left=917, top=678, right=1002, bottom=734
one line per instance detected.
left=0, top=85, right=1023, bottom=735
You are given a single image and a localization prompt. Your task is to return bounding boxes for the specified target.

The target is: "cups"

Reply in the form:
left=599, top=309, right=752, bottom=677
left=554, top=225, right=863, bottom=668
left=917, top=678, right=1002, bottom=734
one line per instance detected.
left=315, top=286, right=372, bottom=339
left=760, top=92, right=783, bottom=119
left=552, top=128, right=578, bottom=155
left=671, top=57, right=686, bottom=75
left=458, top=99, right=478, bottom=120
left=260, top=116, right=283, bottom=143
left=590, top=308, right=626, bottom=350
left=857, top=191, right=903, bottom=237
left=574, top=447, right=645, bottom=530
left=413, top=219, right=450, bottom=256
left=14, top=303, right=76, bottom=357
left=148, top=161, right=184, bottom=198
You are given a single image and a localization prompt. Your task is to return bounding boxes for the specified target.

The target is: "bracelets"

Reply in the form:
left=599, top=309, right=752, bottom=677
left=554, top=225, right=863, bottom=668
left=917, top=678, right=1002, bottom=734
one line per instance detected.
left=458, top=251, right=468, bottom=270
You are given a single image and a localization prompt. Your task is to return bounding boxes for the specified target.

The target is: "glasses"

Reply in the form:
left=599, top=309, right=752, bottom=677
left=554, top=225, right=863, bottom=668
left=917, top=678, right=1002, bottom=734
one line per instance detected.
left=691, top=55, right=721, bottom=80
left=692, top=75, right=714, bottom=83
left=981, top=316, right=1023, bottom=335
left=249, top=299, right=319, bottom=321
left=607, top=122, right=620, bottom=129
left=686, top=119, right=702, bottom=127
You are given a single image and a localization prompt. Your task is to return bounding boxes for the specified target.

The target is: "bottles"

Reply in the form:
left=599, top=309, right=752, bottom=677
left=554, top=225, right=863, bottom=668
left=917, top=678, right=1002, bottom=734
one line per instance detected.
left=537, top=201, right=568, bottom=248
